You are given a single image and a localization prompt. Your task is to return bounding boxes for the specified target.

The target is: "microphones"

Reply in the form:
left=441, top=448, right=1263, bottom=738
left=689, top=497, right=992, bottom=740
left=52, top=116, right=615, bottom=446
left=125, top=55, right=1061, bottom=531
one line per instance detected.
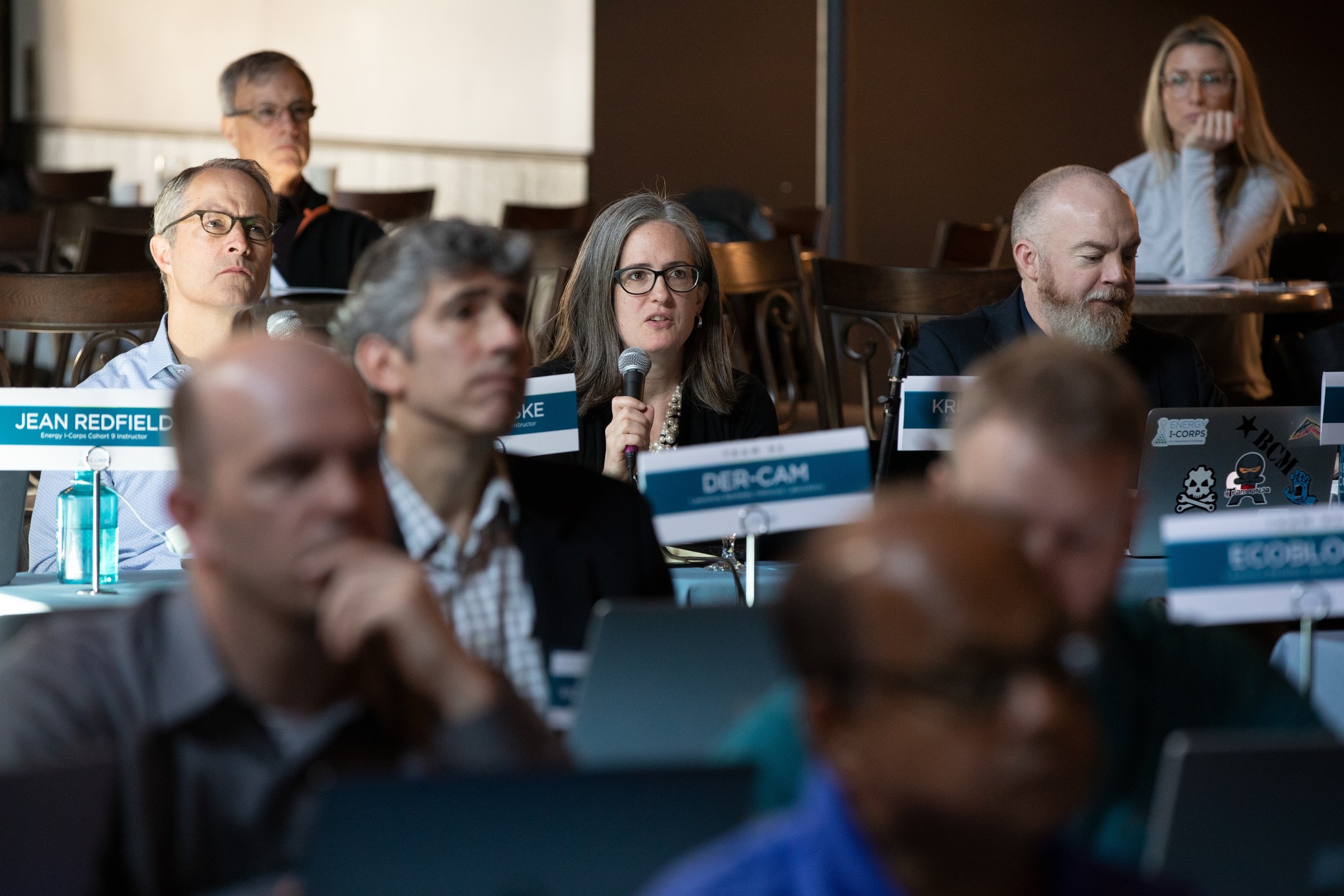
left=616, top=347, right=652, bottom=470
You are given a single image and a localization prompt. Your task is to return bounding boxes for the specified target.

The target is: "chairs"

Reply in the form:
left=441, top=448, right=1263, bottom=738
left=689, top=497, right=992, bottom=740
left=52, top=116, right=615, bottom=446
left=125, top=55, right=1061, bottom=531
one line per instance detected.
left=0, top=171, right=1343, bottom=894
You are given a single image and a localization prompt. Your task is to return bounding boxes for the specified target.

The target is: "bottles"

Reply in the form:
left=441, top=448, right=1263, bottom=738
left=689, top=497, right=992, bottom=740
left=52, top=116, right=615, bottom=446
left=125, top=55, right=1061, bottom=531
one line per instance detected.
left=56, top=471, right=119, bottom=585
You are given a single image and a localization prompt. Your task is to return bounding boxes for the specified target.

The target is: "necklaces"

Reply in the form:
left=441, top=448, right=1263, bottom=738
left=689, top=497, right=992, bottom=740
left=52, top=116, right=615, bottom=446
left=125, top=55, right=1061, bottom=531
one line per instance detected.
left=648, top=381, right=683, bottom=454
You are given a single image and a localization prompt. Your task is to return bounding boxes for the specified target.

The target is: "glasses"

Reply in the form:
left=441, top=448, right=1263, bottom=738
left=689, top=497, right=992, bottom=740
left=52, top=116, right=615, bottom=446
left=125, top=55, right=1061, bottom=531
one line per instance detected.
left=1161, top=71, right=1235, bottom=100
left=611, top=265, right=704, bottom=295
left=847, top=625, right=1099, bottom=704
left=225, top=100, right=316, bottom=125
left=159, top=210, right=282, bottom=242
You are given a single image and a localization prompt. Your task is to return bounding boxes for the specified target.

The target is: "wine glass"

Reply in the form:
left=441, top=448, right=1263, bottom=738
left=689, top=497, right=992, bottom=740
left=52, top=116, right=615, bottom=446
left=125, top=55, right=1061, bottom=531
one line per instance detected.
left=704, top=535, right=747, bottom=572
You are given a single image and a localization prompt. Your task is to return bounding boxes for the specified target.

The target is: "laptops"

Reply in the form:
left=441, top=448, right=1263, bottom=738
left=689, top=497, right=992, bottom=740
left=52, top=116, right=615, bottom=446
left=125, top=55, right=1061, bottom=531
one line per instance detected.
left=1124, top=406, right=1339, bottom=558
left=1140, top=731, right=1342, bottom=896
left=307, top=601, right=800, bottom=896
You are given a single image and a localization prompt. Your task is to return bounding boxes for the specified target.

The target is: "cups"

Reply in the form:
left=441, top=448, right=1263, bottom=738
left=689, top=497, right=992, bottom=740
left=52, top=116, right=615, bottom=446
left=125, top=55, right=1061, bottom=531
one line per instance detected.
left=122, top=184, right=140, bottom=205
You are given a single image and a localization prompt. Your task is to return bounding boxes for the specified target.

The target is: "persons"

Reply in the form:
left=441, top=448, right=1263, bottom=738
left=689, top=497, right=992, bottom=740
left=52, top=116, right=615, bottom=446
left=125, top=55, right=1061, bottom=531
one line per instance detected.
left=0, top=158, right=1344, bottom=896
left=1107, top=17, right=1312, bottom=398
left=221, top=52, right=385, bottom=303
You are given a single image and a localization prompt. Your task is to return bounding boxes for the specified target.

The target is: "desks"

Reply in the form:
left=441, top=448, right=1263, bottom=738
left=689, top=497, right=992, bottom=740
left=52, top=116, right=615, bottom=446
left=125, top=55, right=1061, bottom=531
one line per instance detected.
left=1270, top=631, right=1343, bottom=741
left=1, top=570, right=185, bottom=615
left=671, top=562, right=794, bottom=607
left=1127, top=277, right=1334, bottom=315
